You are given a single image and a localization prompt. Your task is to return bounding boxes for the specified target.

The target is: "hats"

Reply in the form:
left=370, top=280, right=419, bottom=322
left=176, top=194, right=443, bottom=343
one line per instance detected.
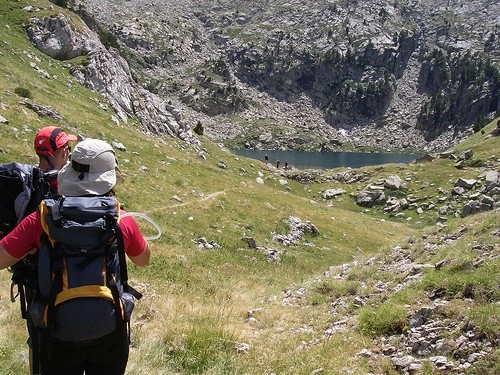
left=57, top=138, right=118, bottom=196
left=33, top=126, right=78, bottom=157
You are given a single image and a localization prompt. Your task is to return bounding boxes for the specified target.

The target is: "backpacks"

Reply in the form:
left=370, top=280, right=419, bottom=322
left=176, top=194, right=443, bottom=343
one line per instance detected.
left=29, top=194, right=142, bottom=345
left=0, top=161, right=39, bottom=272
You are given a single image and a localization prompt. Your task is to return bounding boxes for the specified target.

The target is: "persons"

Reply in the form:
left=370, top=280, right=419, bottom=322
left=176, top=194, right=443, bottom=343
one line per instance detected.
left=265, top=154, right=268, bottom=164
left=26, top=126, right=78, bottom=375
left=0, top=139, right=150, bottom=375
left=285, top=160, right=288, bottom=171
left=276, top=158, right=279, bottom=168
